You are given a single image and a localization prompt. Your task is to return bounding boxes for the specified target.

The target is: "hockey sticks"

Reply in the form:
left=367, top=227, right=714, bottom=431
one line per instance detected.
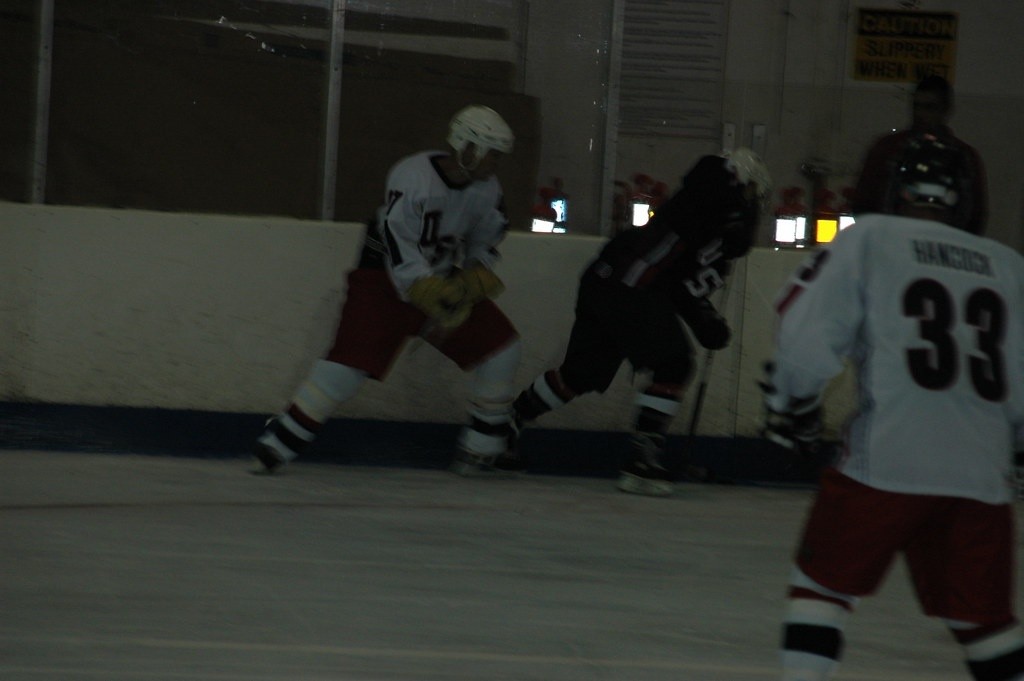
left=685, top=256, right=739, bottom=468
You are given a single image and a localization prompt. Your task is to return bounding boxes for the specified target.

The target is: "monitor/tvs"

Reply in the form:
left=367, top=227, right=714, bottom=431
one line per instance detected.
left=550, top=198, right=568, bottom=223
left=776, top=211, right=855, bottom=248
left=631, top=203, right=652, bottom=228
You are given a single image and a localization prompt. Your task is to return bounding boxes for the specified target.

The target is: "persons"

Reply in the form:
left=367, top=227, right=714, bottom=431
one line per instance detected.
left=770, top=125, right=1024, bottom=681
left=843, top=73, right=988, bottom=237
left=511, top=145, right=773, bottom=498
left=247, top=105, right=521, bottom=477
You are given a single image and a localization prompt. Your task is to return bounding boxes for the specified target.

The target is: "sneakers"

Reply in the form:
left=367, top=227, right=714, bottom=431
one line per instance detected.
left=618, top=456, right=674, bottom=499
left=444, top=442, right=498, bottom=478
left=252, top=418, right=288, bottom=475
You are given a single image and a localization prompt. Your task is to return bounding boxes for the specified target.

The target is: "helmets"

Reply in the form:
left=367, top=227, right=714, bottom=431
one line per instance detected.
left=448, top=105, right=514, bottom=171
left=721, top=147, right=777, bottom=211
left=857, top=134, right=989, bottom=235
